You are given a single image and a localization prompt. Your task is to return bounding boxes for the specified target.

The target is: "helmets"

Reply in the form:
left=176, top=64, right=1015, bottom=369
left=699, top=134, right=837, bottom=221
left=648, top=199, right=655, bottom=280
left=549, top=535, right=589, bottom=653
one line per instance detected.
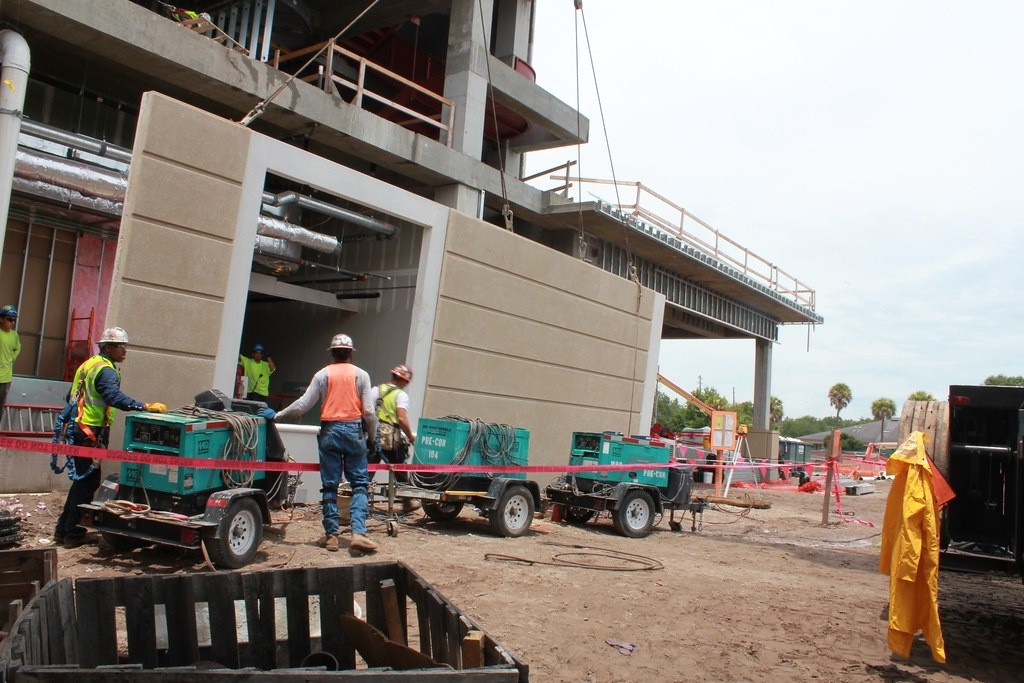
left=390, top=365, right=413, bottom=382
left=98, top=327, right=128, bottom=343
left=253, top=344, right=264, bottom=351
left=327, top=334, right=356, bottom=352
left=198, top=12, right=211, bottom=27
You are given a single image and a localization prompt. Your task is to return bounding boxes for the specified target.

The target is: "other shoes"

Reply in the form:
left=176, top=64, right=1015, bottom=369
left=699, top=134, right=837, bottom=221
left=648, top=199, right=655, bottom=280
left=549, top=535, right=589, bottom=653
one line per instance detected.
left=403, top=498, right=422, bottom=512
left=351, top=533, right=377, bottom=551
left=53, top=531, right=99, bottom=548
left=317, top=532, right=338, bottom=551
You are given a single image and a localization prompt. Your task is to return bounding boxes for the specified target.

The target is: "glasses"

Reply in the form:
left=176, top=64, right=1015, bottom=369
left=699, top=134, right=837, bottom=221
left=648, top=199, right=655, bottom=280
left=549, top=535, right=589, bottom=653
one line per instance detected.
left=0, top=317, right=16, bottom=323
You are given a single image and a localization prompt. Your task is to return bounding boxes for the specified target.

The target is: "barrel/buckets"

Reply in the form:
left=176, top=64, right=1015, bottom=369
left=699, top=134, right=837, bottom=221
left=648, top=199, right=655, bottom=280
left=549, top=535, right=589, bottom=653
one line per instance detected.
left=845, top=486, right=856, bottom=495
left=551, top=504, right=562, bottom=522
left=337, top=488, right=352, bottom=523
left=703, top=472, right=713, bottom=484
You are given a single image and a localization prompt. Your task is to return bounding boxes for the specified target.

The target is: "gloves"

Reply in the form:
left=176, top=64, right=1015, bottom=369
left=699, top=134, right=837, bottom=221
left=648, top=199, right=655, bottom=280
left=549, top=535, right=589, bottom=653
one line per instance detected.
left=366, top=439, right=377, bottom=458
left=256, top=407, right=277, bottom=421
left=143, top=402, right=168, bottom=414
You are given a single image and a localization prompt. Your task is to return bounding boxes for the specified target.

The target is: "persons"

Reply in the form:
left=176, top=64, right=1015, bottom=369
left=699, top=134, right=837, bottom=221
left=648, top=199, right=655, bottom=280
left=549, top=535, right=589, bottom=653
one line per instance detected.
left=367, top=364, right=422, bottom=514
left=237, top=343, right=277, bottom=407
left=53, top=326, right=168, bottom=548
left=170, top=9, right=211, bottom=36
left=0, top=303, right=22, bottom=422
left=256, top=334, right=378, bottom=551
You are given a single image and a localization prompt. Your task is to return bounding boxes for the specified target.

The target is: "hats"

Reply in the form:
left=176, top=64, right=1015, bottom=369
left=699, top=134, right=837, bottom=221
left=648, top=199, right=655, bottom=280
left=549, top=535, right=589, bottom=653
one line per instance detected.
left=0, top=304, right=19, bottom=317
